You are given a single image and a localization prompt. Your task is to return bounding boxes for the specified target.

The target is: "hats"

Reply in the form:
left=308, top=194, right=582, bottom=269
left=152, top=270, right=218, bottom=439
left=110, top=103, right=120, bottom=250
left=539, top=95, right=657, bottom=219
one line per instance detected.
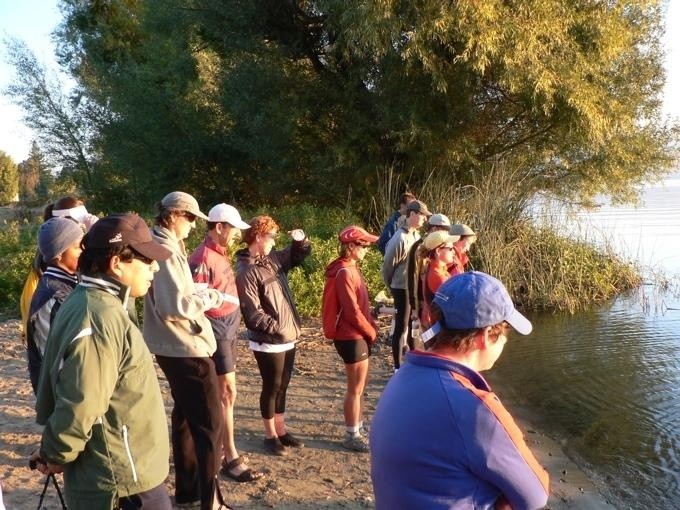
left=406, top=200, right=433, bottom=215
left=432, top=270, right=533, bottom=336
left=48, top=204, right=93, bottom=224
left=428, top=214, right=450, bottom=227
left=338, top=225, right=382, bottom=245
left=446, top=223, right=476, bottom=237
left=161, top=190, right=208, bottom=224
left=38, top=219, right=81, bottom=261
left=423, top=231, right=462, bottom=251
left=83, top=214, right=172, bottom=261
left=208, top=202, right=252, bottom=231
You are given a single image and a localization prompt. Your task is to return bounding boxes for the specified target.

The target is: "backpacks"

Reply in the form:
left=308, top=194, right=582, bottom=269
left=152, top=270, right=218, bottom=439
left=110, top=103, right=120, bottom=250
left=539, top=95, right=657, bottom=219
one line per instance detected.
left=319, top=267, right=353, bottom=339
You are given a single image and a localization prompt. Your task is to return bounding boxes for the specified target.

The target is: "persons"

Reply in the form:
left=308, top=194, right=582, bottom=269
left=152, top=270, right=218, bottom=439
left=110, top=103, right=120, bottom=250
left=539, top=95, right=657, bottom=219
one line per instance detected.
left=30, top=212, right=174, bottom=508
left=370, top=270, right=548, bottom=508
left=231, top=214, right=312, bottom=456
left=20, top=196, right=100, bottom=335
left=27, top=216, right=85, bottom=396
left=375, top=193, right=432, bottom=371
left=407, top=215, right=476, bottom=351
left=144, top=191, right=239, bottom=509
left=188, top=203, right=265, bottom=483
left=322, top=225, right=379, bottom=451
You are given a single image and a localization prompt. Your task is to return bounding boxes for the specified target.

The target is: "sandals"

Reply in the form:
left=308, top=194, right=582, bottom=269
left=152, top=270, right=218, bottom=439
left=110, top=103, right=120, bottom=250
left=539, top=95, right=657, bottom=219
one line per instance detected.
left=220, top=453, right=263, bottom=483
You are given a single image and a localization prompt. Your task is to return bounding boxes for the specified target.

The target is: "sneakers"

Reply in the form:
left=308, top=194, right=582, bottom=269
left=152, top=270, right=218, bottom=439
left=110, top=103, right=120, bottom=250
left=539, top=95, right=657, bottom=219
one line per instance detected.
left=343, top=436, right=372, bottom=453
left=279, top=431, right=306, bottom=448
left=263, top=437, right=287, bottom=457
left=373, top=301, right=394, bottom=345
